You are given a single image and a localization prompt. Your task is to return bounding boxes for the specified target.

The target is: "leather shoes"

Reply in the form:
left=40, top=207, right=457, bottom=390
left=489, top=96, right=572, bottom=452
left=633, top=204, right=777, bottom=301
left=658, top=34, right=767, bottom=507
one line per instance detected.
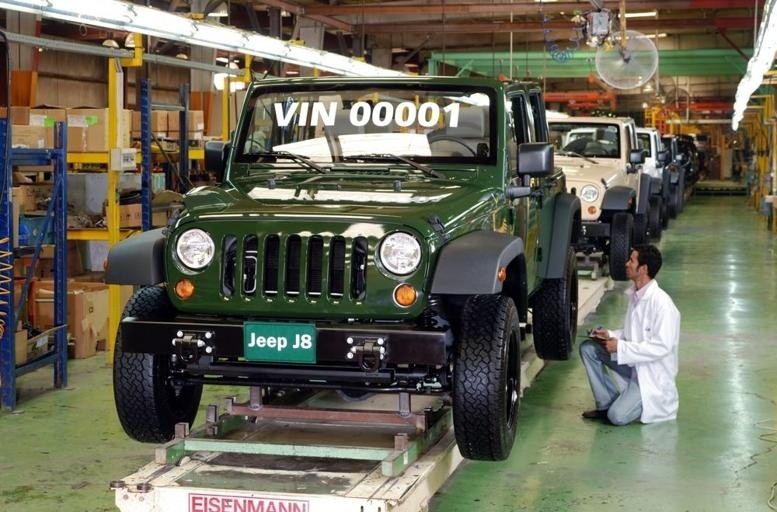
left=584, top=410, right=608, bottom=418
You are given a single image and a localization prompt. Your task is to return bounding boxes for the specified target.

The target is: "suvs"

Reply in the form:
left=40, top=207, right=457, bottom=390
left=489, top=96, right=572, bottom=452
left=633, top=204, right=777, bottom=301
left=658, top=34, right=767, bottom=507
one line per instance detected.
left=547, top=112, right=702, bottom=281
left=113, top=79, right=581, bottom=461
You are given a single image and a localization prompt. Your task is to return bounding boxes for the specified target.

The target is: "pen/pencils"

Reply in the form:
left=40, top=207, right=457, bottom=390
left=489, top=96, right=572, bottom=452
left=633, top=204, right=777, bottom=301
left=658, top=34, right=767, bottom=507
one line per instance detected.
left=591, top=326, right=602, bottom=335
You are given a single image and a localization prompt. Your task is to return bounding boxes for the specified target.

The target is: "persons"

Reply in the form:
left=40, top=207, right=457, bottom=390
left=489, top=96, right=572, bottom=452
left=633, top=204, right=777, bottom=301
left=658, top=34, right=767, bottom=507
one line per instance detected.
left=577, top=243, right=682, bottom=425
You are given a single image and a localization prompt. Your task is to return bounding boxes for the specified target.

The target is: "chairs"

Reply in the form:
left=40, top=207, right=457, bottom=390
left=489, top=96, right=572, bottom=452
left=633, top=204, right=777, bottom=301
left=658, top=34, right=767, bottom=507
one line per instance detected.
left=315, top=107, right=520, bottom=168
left=583, top=127, right=619, bottom=156
